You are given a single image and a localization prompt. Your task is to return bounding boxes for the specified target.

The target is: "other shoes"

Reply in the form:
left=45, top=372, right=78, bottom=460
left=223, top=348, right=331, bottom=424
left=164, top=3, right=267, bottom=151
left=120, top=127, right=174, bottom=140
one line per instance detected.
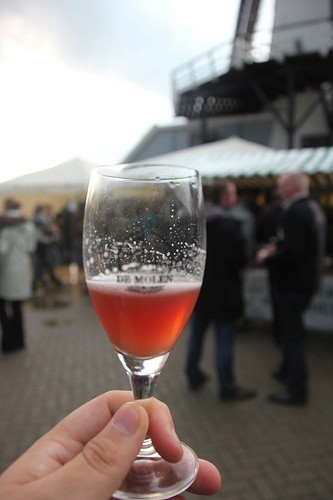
left=274, top=372, right=285, bottom=380
left=270, top=394, right=306, bottom=404
left=232, top=387, right=255, bottom=398
left=199, top=374, right=209, bottom=382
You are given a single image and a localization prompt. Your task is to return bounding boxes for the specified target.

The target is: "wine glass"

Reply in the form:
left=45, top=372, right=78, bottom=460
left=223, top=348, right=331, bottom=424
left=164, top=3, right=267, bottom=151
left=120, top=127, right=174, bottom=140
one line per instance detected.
left=83, top=164, right=207, bottom=500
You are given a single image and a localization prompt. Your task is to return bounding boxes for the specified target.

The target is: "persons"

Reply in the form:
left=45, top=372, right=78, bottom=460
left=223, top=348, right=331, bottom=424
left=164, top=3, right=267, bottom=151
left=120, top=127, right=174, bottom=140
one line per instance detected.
left=185, top=179, right=258, bottom=399
left=0, top=199, right=37, bottom=352
left=257, top=173, right=325, bottom=406
left=32, top=190, right=198, bottom=310
left=249, top=190, right=283, bottom=270
left=0, top=390, right=221, bottom=500
